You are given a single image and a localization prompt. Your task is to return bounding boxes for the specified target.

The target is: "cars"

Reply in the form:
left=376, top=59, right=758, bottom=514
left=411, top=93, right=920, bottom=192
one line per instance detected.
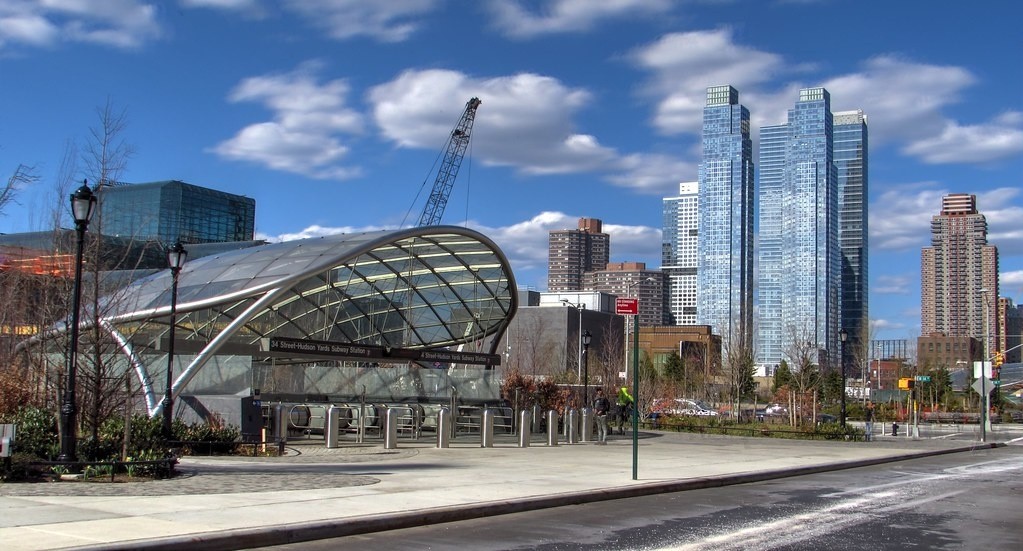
left=765, top=402, right=836, bottom=423
left=662, top=398, right=718, bottom=416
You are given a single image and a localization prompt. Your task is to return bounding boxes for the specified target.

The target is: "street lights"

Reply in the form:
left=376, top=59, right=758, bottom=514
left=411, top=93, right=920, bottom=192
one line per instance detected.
left=154, top=236, right=188, bottom=446
left=582, top=328, right=592, bottom=407
left=560, top=297, right=582, bottom=381
left=838, top=327, right=848, bottom=427
left=57, top=180, right=97, bottom=473
left=980, top=287, right=993, bottom=432
left=624, top=277, right=656, bottom=389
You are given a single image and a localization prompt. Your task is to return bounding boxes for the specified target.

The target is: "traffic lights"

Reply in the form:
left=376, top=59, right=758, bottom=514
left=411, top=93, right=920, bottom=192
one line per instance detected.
left=898, top=377, right=915, bottom=391
left=997, top=352, right=1003, bottom=366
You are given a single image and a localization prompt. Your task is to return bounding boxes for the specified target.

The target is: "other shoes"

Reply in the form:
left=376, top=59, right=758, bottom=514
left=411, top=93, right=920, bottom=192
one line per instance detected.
left=596, top=441, right=607, bottom=445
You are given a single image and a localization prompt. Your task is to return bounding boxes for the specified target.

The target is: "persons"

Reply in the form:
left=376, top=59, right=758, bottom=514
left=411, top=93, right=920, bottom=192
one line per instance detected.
left=594, top=388, right=610, bottom=445
